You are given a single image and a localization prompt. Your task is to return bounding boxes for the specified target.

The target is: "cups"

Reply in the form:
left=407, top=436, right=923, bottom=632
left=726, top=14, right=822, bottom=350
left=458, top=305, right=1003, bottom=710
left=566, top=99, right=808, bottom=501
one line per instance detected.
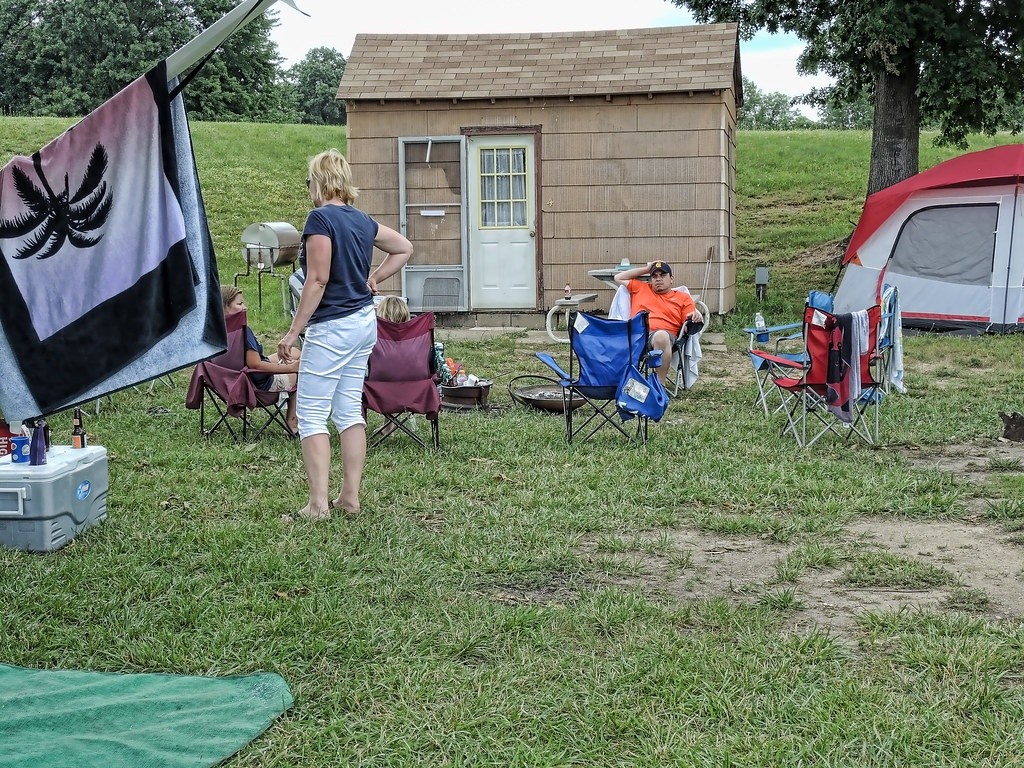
left=10, top=436, right=31, bottom=463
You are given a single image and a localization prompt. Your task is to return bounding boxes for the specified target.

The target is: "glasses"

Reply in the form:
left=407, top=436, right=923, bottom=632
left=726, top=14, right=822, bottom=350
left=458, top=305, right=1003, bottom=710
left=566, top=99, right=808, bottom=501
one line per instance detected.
left=306, top=177, right=310, bottom=188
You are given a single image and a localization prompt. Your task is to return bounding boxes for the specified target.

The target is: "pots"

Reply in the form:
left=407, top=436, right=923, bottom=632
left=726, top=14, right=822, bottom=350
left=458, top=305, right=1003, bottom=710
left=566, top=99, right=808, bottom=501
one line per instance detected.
left=515, top=385, right=590, bottom=411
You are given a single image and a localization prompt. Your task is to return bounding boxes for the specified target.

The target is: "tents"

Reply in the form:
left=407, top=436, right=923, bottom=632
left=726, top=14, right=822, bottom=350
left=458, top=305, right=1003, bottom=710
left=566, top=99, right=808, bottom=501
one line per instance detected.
left=829, top=143, right=1024, bottom=332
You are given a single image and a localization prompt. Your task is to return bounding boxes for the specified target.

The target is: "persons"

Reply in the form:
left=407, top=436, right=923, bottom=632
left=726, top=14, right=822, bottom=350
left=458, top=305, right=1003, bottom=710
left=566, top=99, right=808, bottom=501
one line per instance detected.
left=277, top=147, right=414, bottom=525
left=220, top=283, right=302, bottom=435
left=372, top=294, right=411, bottom=439
left=614, top=260, right=703, bottom=387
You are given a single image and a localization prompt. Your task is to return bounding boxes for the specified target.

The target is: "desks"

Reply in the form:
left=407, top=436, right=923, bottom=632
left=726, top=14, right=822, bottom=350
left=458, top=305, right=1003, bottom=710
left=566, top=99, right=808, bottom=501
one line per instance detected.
left=588, top=268, right=652, bottom=291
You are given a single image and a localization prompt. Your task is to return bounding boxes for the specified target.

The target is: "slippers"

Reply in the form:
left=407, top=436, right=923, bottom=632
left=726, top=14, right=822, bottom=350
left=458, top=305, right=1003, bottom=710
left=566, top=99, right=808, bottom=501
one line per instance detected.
left=374, top=427, right=394, bottom=438
left=329, top=499, right=358, bottom=519
left=277, top=512, right=304, bottom=522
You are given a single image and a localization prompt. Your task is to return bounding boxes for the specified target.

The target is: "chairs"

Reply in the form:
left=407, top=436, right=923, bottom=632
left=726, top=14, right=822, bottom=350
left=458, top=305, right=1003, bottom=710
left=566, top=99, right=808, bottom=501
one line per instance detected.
left=362, top=311, right=441, bottom=455
left=750, top=303, right=884, bottom=446
left=533, top=309, right=666, bottom=445
left=741, top=283, right=897, bottom=408
left=187, top=309, right=298, bottom=442
left=613, top=283, right=704, bottom=398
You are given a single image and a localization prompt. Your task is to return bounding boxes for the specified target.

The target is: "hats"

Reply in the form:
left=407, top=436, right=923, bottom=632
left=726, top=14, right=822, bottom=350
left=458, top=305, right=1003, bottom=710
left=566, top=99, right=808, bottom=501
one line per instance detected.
left=649, top=262, right=671, bottom=276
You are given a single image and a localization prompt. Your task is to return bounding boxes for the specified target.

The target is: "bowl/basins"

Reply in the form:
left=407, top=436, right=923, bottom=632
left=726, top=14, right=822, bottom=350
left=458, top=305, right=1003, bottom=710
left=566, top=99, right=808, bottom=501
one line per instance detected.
left=443, top=378, right=493, bottom=407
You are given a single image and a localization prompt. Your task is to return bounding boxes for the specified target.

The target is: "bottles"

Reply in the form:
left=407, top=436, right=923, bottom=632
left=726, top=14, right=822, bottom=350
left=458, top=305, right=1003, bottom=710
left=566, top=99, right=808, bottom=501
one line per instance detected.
left=755, top=313, right=766, bottom=332
left=564, top=282, right=571, bottom=300
left=71, top=409, right=87, bottom=449
left=457, top=370, right=467, bottom=386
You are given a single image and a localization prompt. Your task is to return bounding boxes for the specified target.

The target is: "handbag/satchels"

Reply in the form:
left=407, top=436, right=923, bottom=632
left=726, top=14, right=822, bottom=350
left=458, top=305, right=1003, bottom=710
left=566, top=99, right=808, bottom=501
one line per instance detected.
left=615, top=364, right=670, bottom=423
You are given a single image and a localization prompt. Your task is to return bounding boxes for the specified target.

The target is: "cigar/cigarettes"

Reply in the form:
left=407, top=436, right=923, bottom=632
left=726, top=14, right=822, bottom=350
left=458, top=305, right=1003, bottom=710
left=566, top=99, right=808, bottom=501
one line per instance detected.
left=277, top=359, right=283, bottom=365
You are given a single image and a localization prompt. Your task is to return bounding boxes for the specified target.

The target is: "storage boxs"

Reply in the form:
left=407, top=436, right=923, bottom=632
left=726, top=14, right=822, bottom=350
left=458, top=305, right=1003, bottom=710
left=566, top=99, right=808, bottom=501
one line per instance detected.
left=0, top=444, right=109, bottom=552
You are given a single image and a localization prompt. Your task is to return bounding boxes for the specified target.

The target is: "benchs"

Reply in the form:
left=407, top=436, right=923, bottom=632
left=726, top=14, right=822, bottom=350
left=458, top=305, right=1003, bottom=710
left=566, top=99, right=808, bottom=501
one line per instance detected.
left=545, top=293, right=598, bottom=344
